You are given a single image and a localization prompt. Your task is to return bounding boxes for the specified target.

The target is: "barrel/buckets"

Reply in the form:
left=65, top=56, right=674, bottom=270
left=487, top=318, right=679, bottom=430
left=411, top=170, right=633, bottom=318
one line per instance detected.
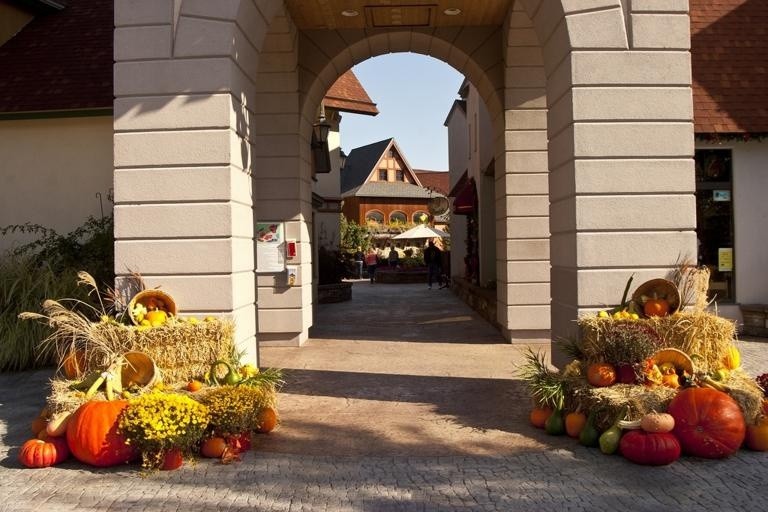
left=120, top=352, right=163, bottom=395
left=739, top=301, right=767, bottom=336
left=649, top=347, right=696, bottom=379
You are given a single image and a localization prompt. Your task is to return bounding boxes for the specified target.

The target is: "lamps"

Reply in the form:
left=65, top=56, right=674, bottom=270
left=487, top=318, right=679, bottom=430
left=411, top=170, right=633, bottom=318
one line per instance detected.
left=309, top=113, right=332, bottom=153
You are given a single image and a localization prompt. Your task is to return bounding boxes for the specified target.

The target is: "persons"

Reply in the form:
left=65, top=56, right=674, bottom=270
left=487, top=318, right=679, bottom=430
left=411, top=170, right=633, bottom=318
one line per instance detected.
left=424, top=242, right=442, bottom=290
left=354, top=247, right=365, bottom=279
left=365, top=248, right=377, bottom=283
left=389, top=246, right=398, bottom=271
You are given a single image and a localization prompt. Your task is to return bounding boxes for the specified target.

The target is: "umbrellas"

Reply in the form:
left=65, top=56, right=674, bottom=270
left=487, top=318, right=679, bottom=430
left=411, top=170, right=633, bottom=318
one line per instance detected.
left=392, top=224, right=450, bottom=247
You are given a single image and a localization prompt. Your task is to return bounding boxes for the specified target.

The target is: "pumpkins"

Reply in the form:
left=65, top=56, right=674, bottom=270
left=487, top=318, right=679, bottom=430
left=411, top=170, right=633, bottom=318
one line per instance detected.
left=528, top=293, right=768, bottom=466
left=20, top=304, right=275, bottom=471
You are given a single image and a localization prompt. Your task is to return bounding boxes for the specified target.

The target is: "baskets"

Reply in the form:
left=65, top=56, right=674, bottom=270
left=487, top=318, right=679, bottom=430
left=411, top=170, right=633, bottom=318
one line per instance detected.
left=643, top=346, right=700, bottom=388
